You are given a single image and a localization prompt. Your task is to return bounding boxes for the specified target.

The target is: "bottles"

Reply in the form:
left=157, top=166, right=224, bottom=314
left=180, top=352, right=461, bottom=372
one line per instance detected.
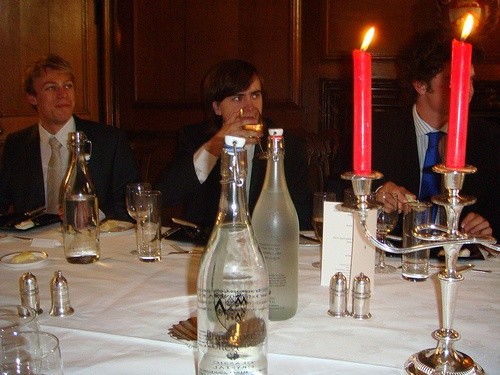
left=62, top=131, right=100, bottom=265
left=252, top=137, right=300, bottom=321
left=19, top=269, right=75, bottom=318
left=196, top=147, right=269, bottom=375
left=327, top=271, right=372, bottom=320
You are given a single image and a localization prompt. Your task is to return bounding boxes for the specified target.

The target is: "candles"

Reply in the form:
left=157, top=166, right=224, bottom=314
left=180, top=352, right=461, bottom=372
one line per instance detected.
left=352, top=26, right=375, bottom=175
left=446, top=12, right=474, bottom=167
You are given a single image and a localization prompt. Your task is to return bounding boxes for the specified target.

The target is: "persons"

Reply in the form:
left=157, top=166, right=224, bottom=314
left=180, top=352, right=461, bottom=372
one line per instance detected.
left=159, top=59, right=315, bottom=231
left=0, top=53, right=138, bottom=223
left=326, top=28, right=500, bottom=244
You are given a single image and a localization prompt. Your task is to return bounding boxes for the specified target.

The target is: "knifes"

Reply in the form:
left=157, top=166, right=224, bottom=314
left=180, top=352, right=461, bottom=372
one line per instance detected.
left=298, top=232, right=320, bottom=243
left=171, top=217, right=207, bottom=236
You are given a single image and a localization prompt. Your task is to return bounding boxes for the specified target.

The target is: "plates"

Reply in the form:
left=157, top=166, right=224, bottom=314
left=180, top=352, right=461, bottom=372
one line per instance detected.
left=0, top=250, right=48, bottom=268
left=163, top=219, right=212, bottom=243
left=0, top=213, right=62, bottom=232
left=100, top=219, right=135, bottom=235
left=299, top=230, right=321, bottom=247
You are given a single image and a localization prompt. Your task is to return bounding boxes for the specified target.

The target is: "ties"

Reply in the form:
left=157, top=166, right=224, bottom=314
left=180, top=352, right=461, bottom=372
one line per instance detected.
left=47, top=136, right=63, bottom=210
left=416, top=130, right=446, bottom=238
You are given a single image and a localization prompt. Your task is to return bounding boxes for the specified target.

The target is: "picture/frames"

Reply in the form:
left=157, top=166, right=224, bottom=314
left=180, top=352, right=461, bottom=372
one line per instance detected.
left=320, top=0, right=500, bottom=62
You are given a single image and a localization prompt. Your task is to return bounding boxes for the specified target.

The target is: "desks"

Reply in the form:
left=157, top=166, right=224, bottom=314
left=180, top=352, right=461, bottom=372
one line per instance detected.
left=0, top=221, right=500, bottom=375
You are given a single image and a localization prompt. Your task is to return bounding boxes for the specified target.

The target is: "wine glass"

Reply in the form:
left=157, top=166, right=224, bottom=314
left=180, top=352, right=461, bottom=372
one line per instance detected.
left=239, top=106, right=269, bottom=160
left=367, top=191, right=396, bottom=273
left=125, top=184, right=154, bottom=255
left=312, top=192, right=337, bottom=267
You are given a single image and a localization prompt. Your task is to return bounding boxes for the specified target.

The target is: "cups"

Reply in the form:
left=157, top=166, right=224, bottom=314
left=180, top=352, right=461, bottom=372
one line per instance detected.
left=345, top=190, right=355, bottom=203
left=136, top=191, right=162, bottom=261
left=401, top=201, right=433, bottom=281
left=1, top=305, right=64, bottom=375
